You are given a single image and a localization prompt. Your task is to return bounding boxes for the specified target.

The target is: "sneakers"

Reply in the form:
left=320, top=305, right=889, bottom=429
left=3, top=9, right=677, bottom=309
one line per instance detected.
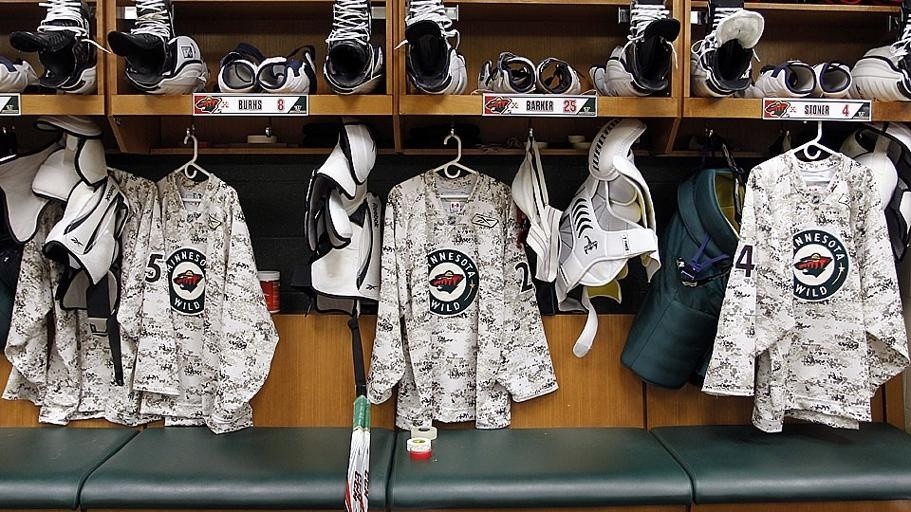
left=690, top=0, right=765, bottom=98
left=321, top=0, right=386, bottom=95
left=394, top=2, right=469, bottom=95
left=846, top=1, right=911, bottom=101
left=624, top=0, right=681, bottom=98
left=107, top=1, right=176, bottom=98
left=9, top=1, right=111, bottom=96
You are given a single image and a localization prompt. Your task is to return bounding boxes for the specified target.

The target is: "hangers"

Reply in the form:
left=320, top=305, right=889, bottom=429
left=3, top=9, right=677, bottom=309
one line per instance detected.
left=175, top=136, right=211, bottom=203
left=793, top=120, right=842, bottom=186
left=433, top=134, right=482, bottom=200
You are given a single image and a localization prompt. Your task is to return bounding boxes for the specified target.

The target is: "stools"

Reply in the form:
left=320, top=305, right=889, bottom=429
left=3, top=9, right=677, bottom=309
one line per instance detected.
left=0, top=426, right=142, bottom=510
left=77, top=426, right=395, bottom=511
left=650, top=420, right=911, bottom=510
left=386, top=427, right=693, bottom=511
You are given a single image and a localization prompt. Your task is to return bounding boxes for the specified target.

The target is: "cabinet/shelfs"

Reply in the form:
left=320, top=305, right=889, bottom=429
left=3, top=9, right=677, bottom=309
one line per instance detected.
left=0, top=1, right=105, bottom=115
left=683, top=2, right=911, bottom=158
left=398, top=1, right=682, bottom=157
left=106, top=1, right=397, bottom=160
